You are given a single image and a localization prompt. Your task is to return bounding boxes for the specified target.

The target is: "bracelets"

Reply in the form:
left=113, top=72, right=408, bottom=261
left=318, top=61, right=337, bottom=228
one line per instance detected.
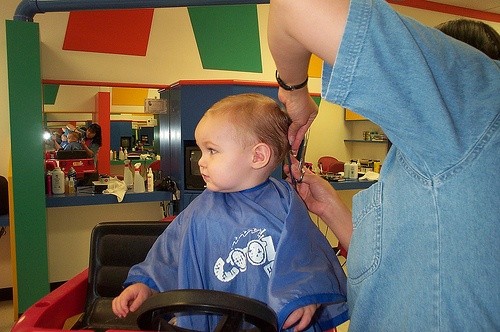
left=74, top=128, right=77, bottom=132
left=276, top=70, right=308, bottom=91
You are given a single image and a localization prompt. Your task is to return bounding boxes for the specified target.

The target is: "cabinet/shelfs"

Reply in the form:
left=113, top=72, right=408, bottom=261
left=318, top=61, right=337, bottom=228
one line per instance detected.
left=169, top=80, right=286, bottom=216
left=308, top=173, right=379, bottom=247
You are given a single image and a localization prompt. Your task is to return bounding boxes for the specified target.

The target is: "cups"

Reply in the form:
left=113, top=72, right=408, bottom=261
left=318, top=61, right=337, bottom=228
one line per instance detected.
left=365, top=131, right=370, bottom=140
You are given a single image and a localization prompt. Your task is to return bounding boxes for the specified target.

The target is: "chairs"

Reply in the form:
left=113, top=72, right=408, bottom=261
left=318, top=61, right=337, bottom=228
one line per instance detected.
left=56, top=150, right=88, bottom=160
left=80, top=221, right=173, bottom=330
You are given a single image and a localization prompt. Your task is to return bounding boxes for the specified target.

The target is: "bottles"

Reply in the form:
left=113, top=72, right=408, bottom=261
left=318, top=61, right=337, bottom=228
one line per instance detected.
left=374, top=160, right=381, bottom=173
left=68, top=167, right=77, bottom=193
left=368, top=160, right=372, bottom=166
left=52, top=167, right=65, bottom=194
left=350, top=162, right=358, bottom=179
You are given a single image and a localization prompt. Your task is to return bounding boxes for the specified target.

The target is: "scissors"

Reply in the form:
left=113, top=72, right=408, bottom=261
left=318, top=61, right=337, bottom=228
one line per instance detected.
left=298, top=128, right=310, bottom=183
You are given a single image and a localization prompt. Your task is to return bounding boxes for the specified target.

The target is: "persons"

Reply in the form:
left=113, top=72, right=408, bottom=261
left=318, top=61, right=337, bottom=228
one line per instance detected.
left=113, top=93, right=351, bottom=332
left=267, top=1, right=500, bottom=331
left=49, top=122, right=103, bottom=160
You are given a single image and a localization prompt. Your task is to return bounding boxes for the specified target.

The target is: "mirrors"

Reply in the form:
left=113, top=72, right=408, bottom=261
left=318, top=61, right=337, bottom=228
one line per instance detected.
left=42, top=81, right=169, bottom=195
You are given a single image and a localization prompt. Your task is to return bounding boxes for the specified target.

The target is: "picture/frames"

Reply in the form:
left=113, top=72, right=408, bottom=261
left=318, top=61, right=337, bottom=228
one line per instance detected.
left=120, top=136, right=131, bottom=150
